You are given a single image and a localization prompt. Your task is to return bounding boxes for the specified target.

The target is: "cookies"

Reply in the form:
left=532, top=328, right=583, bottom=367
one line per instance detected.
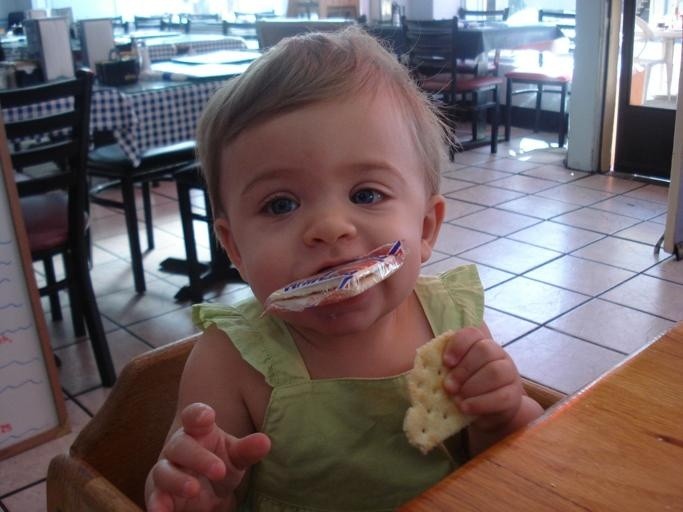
left=268, top=254, right=398, bottom=310
left=401, top=328, right=480, bottom=455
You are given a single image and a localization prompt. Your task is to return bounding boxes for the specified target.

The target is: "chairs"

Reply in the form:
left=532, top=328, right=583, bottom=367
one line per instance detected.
left=504, top=9, right=574, bottom=149
left=0, top=0, right=406, bottom=404
left=400, top=15, right=507, bottom=163
left=416, top=7, right=511, bottom=128
left=45, top=326, right=570, bottom=510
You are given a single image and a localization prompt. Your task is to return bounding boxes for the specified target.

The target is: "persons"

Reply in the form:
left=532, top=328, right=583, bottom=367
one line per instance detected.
left=142, top=26, right=546, bottom=509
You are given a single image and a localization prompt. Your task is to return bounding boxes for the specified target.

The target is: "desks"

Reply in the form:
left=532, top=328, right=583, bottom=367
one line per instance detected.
left=391, top=316, right=683, bottom=511
left=365, top=19, right=566, bottom=152
